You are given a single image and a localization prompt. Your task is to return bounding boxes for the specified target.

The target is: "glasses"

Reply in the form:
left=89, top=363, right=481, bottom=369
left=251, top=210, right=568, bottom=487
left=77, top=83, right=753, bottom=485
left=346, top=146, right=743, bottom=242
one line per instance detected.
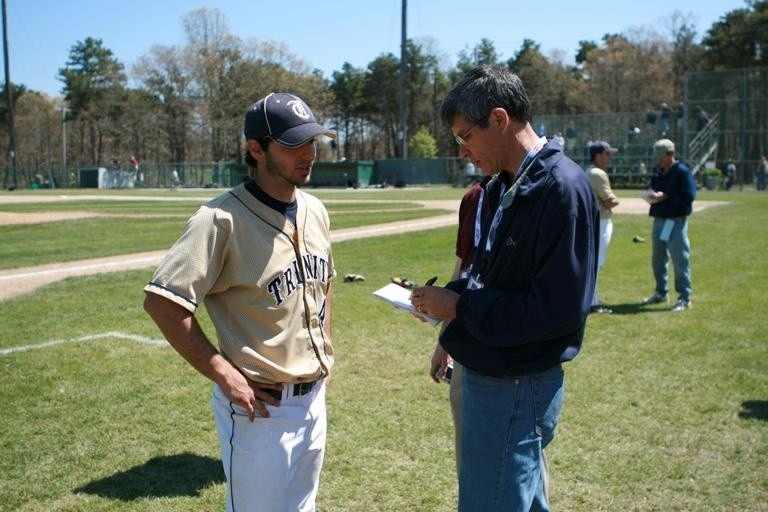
left=454, top=120, right=480, bottom=145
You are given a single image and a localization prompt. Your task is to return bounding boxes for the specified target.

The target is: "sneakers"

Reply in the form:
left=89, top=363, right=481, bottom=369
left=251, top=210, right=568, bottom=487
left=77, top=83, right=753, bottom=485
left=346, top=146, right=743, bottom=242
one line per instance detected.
left=643, top=293, right=669, bottom=305
left=671, top=297, right=692, bottom=312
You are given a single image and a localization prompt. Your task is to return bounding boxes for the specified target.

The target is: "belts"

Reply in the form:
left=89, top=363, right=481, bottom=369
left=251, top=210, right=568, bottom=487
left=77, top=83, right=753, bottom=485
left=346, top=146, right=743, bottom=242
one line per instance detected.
left=258, top=380, right=317, bottom=402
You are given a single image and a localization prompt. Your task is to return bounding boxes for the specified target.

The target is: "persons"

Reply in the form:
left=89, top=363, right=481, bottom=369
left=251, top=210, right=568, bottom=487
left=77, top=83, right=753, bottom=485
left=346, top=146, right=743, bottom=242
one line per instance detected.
left=410, top=62, right=605, bottom=511
left=532, top=98, right=768, bottom=195
left=636, top=138, right=697, bottom=313
left=583, top=139, right=624, bottom=315
left=462, top=159, right=475, bottom=187
left=142, top=91, right=338, bottom=511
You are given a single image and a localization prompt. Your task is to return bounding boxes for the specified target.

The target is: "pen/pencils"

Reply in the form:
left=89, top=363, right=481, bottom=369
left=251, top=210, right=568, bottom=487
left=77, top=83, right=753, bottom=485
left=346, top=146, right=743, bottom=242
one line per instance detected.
left=425, top=276, right=438, bottom=287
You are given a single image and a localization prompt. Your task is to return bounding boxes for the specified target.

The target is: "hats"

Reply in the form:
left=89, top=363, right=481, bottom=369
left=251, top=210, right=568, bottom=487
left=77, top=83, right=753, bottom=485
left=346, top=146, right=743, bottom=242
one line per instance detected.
left=246, top=92, right=338, bottom=149
left=651, top=138, right=676, bottom=160
left=590, top=141, right=618, bottom=155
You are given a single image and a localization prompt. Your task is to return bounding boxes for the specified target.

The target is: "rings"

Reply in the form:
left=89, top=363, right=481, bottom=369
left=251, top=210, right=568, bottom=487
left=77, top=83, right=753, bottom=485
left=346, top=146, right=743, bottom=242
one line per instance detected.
left=418, top=306, right=421, bottom=312
